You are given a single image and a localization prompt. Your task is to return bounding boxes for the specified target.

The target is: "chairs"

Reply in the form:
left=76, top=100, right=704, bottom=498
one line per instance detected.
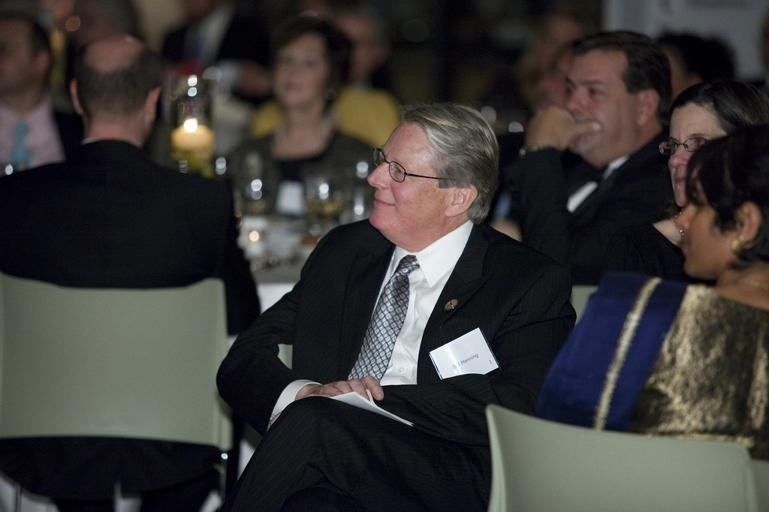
left=0, top=273, right=238, bottom=511
left=570, top=280, right=597, bottom=318
left=483, top=401, right=761, bottom=512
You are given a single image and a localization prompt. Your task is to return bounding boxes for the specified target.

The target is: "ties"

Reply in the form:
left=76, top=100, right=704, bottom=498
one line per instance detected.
left=346, top=254, right=420, bottom=386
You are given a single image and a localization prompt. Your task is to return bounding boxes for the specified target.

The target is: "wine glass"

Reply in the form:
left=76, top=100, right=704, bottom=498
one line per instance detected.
left=301, top=173, right=350, bottom=237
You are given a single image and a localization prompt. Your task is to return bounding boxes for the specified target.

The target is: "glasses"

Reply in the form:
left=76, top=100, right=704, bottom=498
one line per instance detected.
left=370, top=147, right=458, bottom=184
left=658, top=136, right=709, bottom=157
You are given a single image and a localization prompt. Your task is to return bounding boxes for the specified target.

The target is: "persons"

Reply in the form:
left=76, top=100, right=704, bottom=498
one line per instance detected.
left=217, top=103, right=577, bottom=511
left=1, top=0, right=403, bottom=512
left=490, top=12, right=768, bottom=311
